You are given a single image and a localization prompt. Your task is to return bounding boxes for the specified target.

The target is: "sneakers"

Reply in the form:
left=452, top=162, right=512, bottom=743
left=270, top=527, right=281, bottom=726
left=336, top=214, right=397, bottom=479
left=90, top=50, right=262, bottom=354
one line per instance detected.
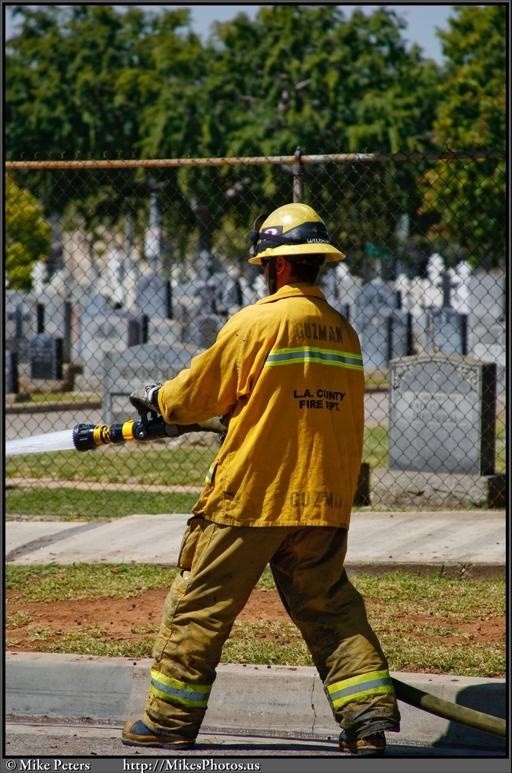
left=338, top=729, right=388, bottom=758
left=121, top=717, right=198, bottom=751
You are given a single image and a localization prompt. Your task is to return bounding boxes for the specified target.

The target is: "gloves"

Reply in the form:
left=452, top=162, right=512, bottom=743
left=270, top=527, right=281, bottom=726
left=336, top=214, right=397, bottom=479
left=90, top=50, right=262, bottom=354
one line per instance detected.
left=142, top=381, right=161, bottom=411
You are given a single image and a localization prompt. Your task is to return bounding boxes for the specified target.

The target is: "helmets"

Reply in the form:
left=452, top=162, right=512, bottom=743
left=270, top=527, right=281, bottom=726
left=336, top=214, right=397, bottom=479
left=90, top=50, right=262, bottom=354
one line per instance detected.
left=248, top=204, right=347, bottom=262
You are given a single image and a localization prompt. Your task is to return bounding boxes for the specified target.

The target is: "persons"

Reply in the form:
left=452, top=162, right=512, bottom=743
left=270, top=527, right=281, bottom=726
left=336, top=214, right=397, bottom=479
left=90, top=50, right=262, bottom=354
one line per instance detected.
left=120, top=202, right=400, bottom=757
